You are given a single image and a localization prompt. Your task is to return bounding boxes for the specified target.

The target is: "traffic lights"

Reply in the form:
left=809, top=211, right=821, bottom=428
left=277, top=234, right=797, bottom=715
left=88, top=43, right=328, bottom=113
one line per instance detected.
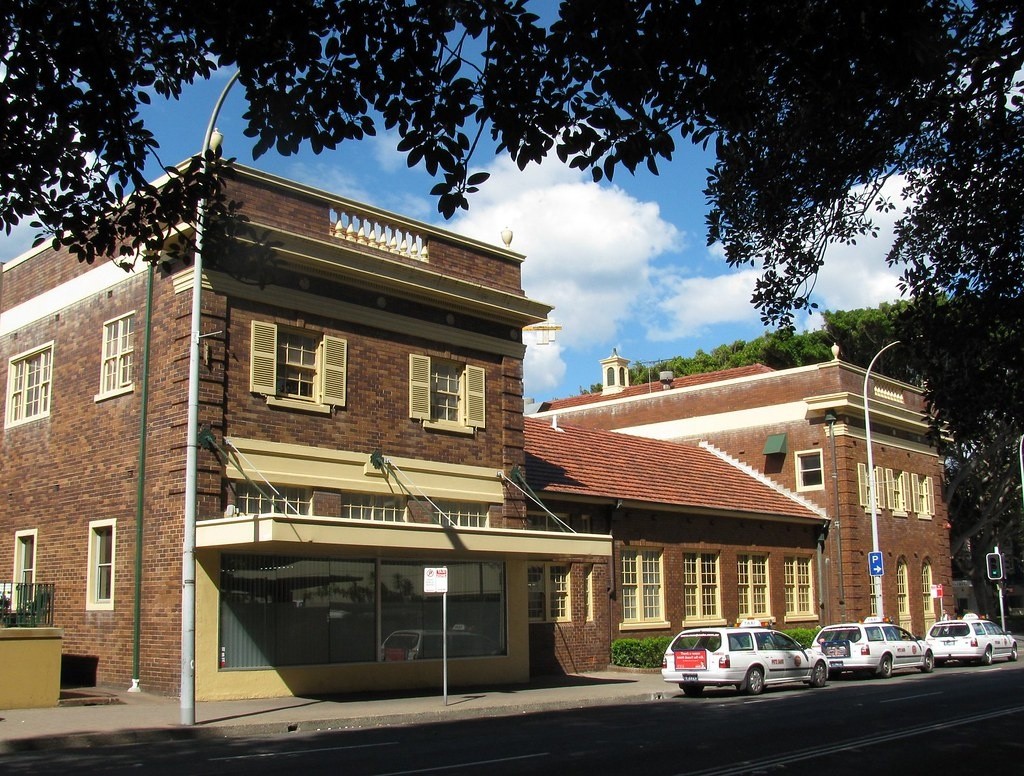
left=986, top=552, right=1003, bottom=579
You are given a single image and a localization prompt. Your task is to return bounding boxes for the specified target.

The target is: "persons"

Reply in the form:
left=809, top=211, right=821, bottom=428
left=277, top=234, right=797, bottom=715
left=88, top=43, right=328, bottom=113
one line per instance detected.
left=941, top=610, right=951, bottom=634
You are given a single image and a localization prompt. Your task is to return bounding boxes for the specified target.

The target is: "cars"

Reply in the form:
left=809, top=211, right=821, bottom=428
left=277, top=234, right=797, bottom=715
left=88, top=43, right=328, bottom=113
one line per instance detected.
left=659, top=618, right=831, bottom=697
left=809, top=615, right=936, bottom=680
left=222, top=581, right=506, bottom=661
left=926, top=618, right=1018, bottom=666
